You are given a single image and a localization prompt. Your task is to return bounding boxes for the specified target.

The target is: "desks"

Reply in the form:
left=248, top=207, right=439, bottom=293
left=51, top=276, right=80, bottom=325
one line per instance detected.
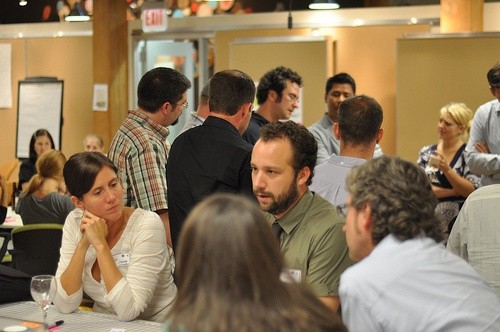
left=0, top=300, right=162, bottom=332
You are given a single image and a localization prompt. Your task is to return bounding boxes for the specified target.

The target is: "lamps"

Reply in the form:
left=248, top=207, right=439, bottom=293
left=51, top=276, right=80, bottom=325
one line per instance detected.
left=64, top=0, right=90, bottom=22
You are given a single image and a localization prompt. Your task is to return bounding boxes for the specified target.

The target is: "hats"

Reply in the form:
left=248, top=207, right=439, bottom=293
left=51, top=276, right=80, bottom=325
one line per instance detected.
left=487, top=68, right=500, bottom=87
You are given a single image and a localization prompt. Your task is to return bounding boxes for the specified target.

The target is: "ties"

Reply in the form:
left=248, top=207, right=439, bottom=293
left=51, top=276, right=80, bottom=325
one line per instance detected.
left=271, top=223, right=284, bottom=248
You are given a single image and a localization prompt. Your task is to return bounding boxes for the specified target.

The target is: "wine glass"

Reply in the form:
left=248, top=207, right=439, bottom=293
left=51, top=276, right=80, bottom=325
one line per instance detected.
left=30, top=274, right=57, bottom=332
left=427, top=151, right=440, bottom=183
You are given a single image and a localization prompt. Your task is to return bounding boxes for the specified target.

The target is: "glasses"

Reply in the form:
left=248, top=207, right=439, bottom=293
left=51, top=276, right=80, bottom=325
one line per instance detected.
left=336, top=203, right=352, bottom=218
left=172, top=101, right=188, bottom=109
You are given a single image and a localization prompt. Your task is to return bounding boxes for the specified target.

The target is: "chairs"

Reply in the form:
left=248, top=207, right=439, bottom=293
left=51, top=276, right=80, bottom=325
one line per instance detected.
left=11, top=223, right=63, bottom=276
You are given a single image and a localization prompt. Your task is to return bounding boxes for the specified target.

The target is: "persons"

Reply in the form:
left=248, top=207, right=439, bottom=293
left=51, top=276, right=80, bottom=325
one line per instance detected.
left=248, top=120, right=357, bottom=313
left=306, top=72, right=384, bottom=165
left=416, top=102, right=484, bottom=247
left=0, top=129, right=107, bottom=224
left=161, top=192, right=349, bottom=332
left=48, top=152, right=179, bottom=324
left=104, top=67, right=192, bottom=248
left=241, top=66, right=304, bottom=146
left=464, top=63, right=500, bottom=186
left=179, top=79, right=209, bottom=133
left=338, top=154, right=500, bottom=332
left=446, top=184, right=500, bottom=300
left=166, top=70, right=254, bottom=250
left=307, top=94, right=384, bottom=207
